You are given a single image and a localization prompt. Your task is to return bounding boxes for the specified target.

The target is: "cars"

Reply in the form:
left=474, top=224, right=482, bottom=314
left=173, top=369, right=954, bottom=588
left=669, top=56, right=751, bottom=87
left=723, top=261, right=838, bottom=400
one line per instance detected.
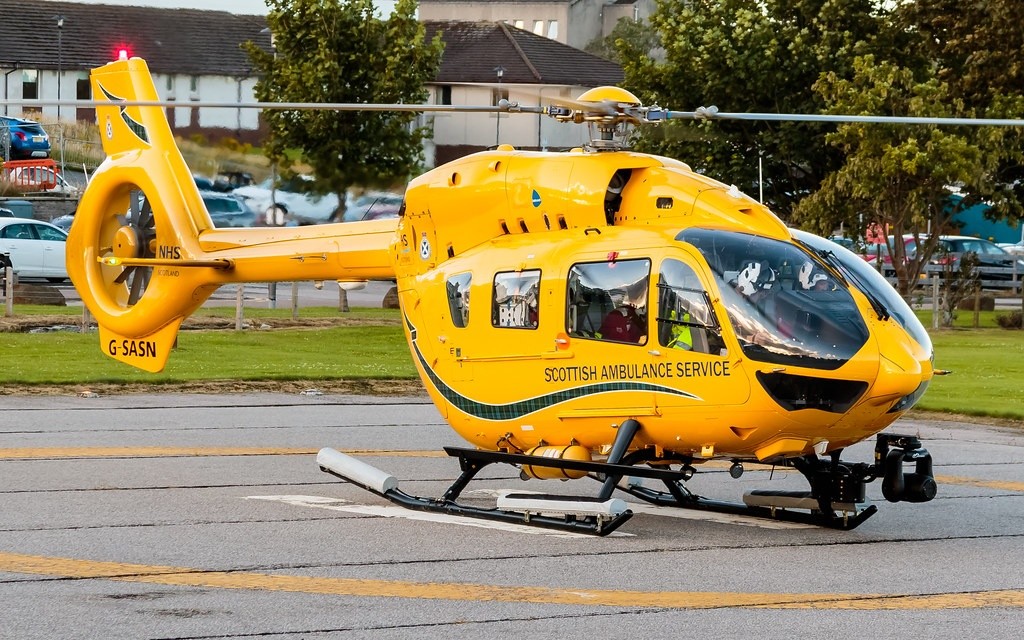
left=192, top=169, right=406, bottom=228
left=829, top=234, right=1024, bottom=296
left=0, top=218, right=73, bottom=284
left=11, top=166, right=77, bottom=194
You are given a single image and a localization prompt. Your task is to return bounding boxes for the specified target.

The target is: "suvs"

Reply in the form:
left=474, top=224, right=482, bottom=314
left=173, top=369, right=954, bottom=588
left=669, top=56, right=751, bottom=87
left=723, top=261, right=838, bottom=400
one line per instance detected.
left=1, top=116, right=51, bottom=160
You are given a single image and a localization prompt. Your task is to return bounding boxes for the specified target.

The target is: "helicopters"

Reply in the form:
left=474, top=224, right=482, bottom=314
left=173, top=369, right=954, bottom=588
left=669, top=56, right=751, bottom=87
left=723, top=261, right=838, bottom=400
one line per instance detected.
left=1, top=46, right=1024, bottom=539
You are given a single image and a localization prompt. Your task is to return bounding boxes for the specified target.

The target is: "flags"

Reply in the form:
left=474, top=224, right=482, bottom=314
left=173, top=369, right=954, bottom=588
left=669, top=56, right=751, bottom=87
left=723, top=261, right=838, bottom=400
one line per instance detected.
left=866, top=221, right=889, bottom=244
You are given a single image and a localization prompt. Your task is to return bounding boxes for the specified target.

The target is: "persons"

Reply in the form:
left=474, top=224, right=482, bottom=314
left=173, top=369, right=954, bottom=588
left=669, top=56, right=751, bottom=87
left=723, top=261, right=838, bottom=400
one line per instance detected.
left=706, top=261, right=776, bottom=356
left=668, top=300, right=693, bottom=352
left=600, top=304, right=648, bottom=345
left=529, top=293, right=538, bottom=328
left=798, top=261, right=836, bottom=291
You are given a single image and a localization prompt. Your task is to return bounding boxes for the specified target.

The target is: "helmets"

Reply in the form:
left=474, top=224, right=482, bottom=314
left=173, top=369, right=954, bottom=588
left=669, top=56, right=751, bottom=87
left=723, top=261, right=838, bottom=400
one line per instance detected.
left=799, top=258, right=837, bottom=291
left=737, top=262, right=775, bottom=296
